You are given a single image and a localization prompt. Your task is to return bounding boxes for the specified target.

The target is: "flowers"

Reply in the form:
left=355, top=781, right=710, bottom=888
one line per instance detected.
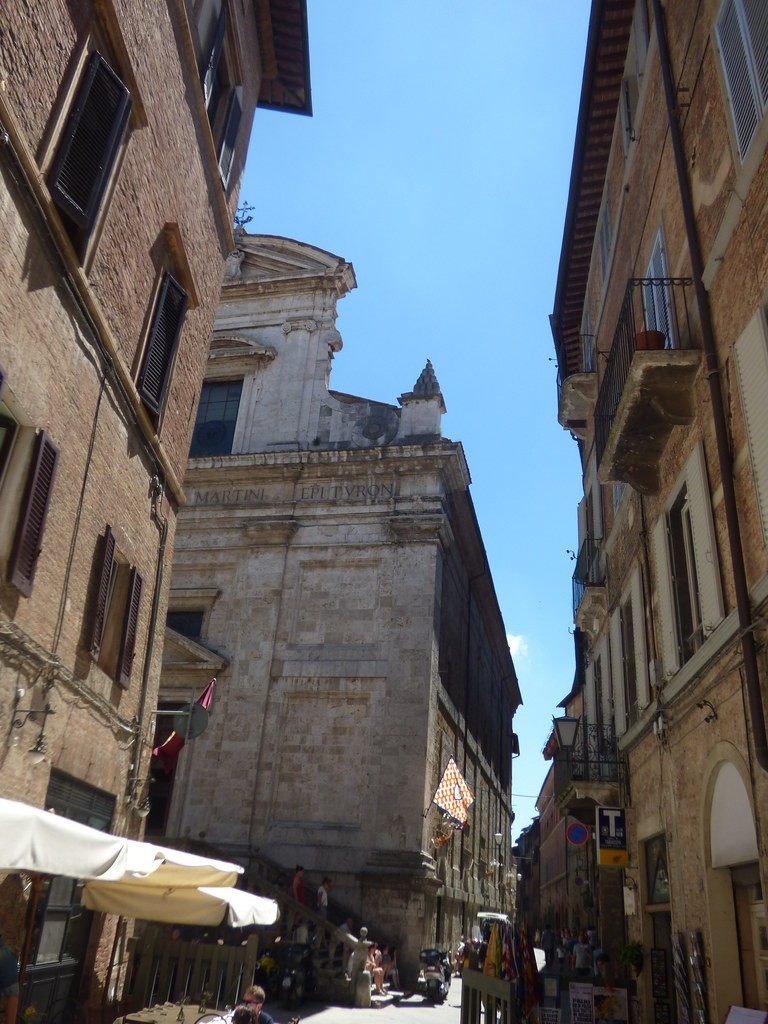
left=200, top=992, right=211, bottom=1003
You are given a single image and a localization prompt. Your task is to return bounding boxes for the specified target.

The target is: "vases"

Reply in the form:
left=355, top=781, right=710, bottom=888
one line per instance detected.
left=198, top=1003, right=206, bottom=1013
left=636, top=331, right=666, bottom=350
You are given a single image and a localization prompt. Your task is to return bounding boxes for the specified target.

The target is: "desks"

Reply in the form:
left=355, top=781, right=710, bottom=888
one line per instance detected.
left=114, top=1004, right=227, bottom=1024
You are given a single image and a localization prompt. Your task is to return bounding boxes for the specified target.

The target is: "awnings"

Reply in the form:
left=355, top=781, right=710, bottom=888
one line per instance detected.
left=0, top=799, right=282, bottom=927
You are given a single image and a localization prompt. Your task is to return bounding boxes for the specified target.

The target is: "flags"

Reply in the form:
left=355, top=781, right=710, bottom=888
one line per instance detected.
left=432, top=755, right=476, bottom=826
left=482, top=918, right=542, bottom=1020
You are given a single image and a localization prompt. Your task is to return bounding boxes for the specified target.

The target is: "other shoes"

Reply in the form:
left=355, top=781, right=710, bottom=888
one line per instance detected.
left=374, top=989, right=385, bottom=996
left=380, top=988, right=387, bottom=995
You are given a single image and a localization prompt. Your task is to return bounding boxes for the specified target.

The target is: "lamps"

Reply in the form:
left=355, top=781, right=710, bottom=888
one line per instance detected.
left=126, top=776, right=156, bottom=817
left=485, top=833, right=504, bottom=849
left=6, top=701, right=56, bottom=766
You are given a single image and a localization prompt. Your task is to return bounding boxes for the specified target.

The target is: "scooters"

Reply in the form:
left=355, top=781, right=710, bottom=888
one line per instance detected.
left=418, top=941, right=456, bottom=1004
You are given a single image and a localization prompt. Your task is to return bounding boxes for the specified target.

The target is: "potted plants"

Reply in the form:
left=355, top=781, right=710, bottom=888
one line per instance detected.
left=174, top=997, right=190, bottom=1020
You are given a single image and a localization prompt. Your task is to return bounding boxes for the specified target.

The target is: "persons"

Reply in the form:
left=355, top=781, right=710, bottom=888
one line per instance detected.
left=230, top=985, right=274, bottom=1024
left=534, top=922, right=598, bottom=981
left=310, top=878, right=332, bottom=946
left=336, top=918, right=403, bottom=995
left=0, top=930, right=20, bottom=1024
left=289, top=865, right=308, bottom=927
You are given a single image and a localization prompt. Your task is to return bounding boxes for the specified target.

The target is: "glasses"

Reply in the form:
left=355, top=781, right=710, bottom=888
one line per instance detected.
left=241, top=999, right=260, bottom=1004
left=231, top=1017, right=236, bottom=1022
